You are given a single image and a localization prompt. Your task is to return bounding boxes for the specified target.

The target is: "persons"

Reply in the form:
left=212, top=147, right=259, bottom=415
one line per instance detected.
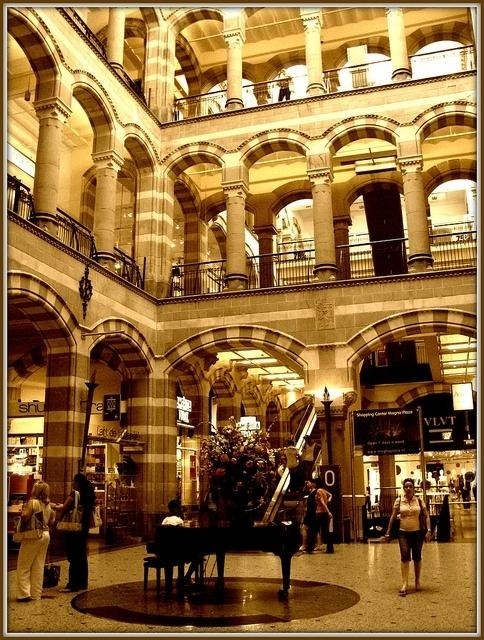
left=12, top=481, right=55, bottom=602
left=427, top=470, right=450, bottom=516
left=50, top=473, right=95, bottom=593
left=460, top=472, right=477, bottom=509
left=160, top=498, right=184, bottom=528
left=171, top=256, right=184, bottom=296
left=298, top=478, right=334, bottom=555
left=384, top=478, right=432, bottom=596
left=276, top=68, right=291, bottom=102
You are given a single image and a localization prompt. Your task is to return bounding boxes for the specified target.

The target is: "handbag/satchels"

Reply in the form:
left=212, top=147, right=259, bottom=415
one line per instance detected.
left=418, top=497, right=428, bottom=536
left=89, top=505, right=103, bottom=529
left=13, top=499, right=43, bottom=542
left=55, top=490, right=83, bottom=532
left=42, top=562, right=61, bottom=588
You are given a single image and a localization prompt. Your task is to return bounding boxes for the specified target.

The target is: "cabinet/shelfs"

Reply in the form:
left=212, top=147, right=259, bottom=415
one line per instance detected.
left=7, top=443, right=119, bottom=538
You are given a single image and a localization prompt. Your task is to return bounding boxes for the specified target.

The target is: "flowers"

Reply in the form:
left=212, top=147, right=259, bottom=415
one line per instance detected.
left=187, top=415, right=288, bottom=511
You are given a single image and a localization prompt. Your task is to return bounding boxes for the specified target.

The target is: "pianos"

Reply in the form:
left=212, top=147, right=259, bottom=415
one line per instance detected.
left=156, top=522, right=302, bottom=602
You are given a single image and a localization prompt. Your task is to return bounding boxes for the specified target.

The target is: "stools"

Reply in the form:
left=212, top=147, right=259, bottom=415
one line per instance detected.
left=143, top=557, right=206, bottom=598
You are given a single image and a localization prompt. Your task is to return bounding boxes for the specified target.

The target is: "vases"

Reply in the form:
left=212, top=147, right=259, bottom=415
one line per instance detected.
left=216, top=492, right=250, bottom=518
